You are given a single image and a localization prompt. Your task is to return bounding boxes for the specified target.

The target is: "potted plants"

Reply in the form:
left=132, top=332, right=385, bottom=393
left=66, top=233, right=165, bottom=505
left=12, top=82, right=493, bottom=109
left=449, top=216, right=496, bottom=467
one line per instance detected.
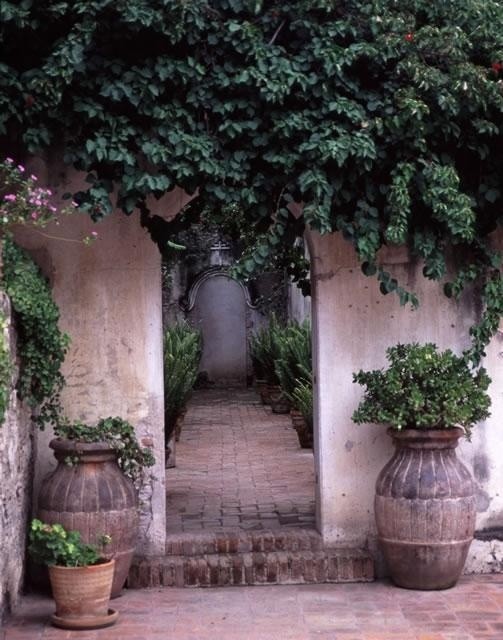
left=245, top=312, right=316, bottom=452
left=347, top=333, right=493, bottom=591
left=26, top=321, right=203, bottom=631
